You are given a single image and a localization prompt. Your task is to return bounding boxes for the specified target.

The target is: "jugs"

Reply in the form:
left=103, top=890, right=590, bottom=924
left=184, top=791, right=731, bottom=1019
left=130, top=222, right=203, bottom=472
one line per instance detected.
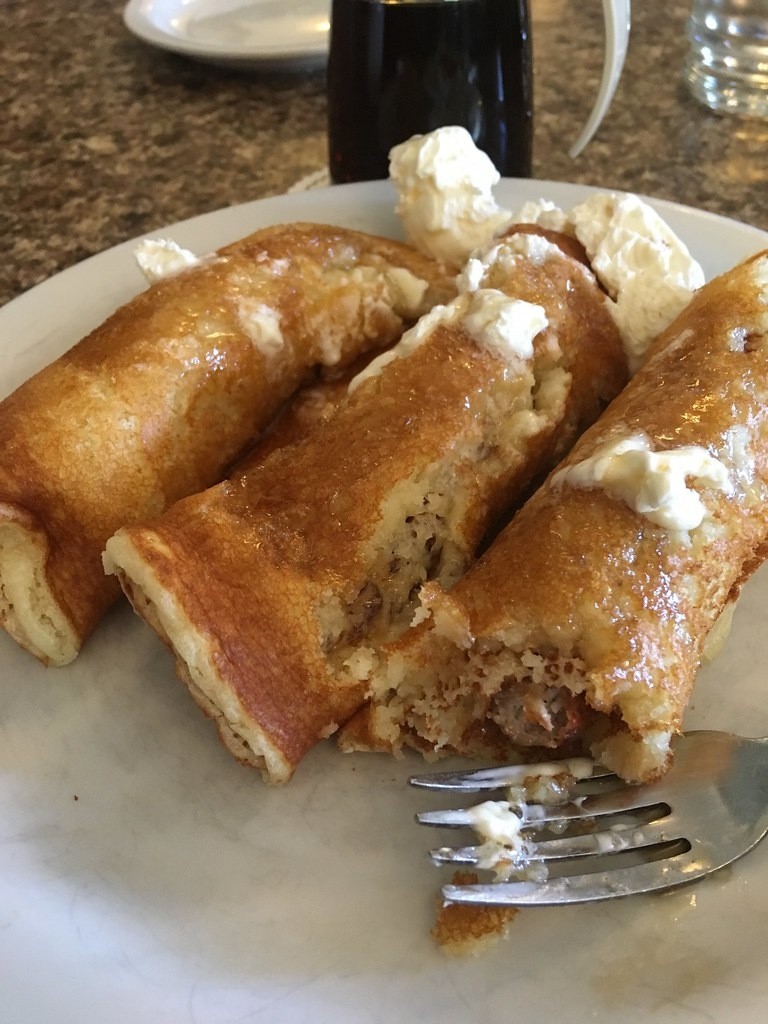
left=330, top=0, right=631, bottom=187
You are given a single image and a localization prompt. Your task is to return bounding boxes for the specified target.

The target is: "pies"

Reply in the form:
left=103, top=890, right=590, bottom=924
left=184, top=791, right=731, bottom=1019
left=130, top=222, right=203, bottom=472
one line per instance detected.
left=0, top=220, right=768, bottom=785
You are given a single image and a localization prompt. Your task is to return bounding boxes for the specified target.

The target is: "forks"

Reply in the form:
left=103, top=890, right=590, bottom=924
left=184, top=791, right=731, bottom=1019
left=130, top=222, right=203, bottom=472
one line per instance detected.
left=408, top=729, right=768, bottom=906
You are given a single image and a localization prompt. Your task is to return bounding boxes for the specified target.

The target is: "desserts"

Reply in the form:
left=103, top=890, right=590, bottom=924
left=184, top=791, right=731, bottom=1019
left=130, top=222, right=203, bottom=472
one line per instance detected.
left=387, top=128, right=706, bottom=361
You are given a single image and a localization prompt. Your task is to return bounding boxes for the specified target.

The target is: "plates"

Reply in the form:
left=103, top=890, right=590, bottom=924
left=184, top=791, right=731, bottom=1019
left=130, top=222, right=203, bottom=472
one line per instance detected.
left=0, top=177, right=768, bottom=1024
left=124, top=0, right=337, bottom=74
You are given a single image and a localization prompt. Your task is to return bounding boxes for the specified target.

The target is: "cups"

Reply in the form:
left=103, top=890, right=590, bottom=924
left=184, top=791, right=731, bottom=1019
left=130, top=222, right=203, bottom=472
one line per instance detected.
left=685, top=0, right=768, bottom=118
